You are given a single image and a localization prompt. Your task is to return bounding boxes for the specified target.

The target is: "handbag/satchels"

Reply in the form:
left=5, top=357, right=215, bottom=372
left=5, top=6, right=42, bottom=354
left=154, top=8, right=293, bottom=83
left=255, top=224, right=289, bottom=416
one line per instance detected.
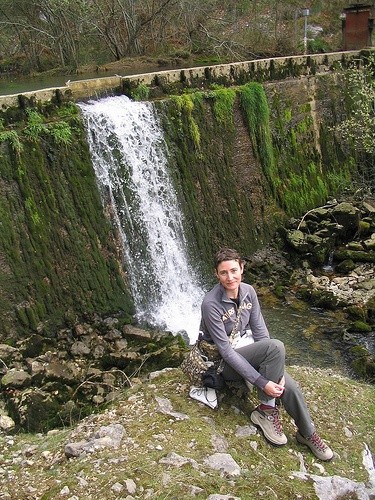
left=180, top=340, right=226, bottom=410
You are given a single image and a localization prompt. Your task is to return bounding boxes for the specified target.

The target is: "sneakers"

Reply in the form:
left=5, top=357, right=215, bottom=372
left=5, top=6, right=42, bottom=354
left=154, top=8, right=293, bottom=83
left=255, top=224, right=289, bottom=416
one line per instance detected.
left=250, top=405, right=288, bottom=445
left=296, top=430, right=334, bottom=460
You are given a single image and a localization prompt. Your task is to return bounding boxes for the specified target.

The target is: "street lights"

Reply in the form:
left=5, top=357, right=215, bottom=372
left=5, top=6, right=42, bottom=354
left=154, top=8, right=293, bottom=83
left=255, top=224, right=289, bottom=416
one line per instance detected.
left=302, top=7, right=310, bottom=54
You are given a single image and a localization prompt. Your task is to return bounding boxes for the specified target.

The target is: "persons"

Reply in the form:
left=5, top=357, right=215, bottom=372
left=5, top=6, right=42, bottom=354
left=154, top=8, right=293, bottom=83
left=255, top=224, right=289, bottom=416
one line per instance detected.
left=197, top=248, right=334, bottom=461
